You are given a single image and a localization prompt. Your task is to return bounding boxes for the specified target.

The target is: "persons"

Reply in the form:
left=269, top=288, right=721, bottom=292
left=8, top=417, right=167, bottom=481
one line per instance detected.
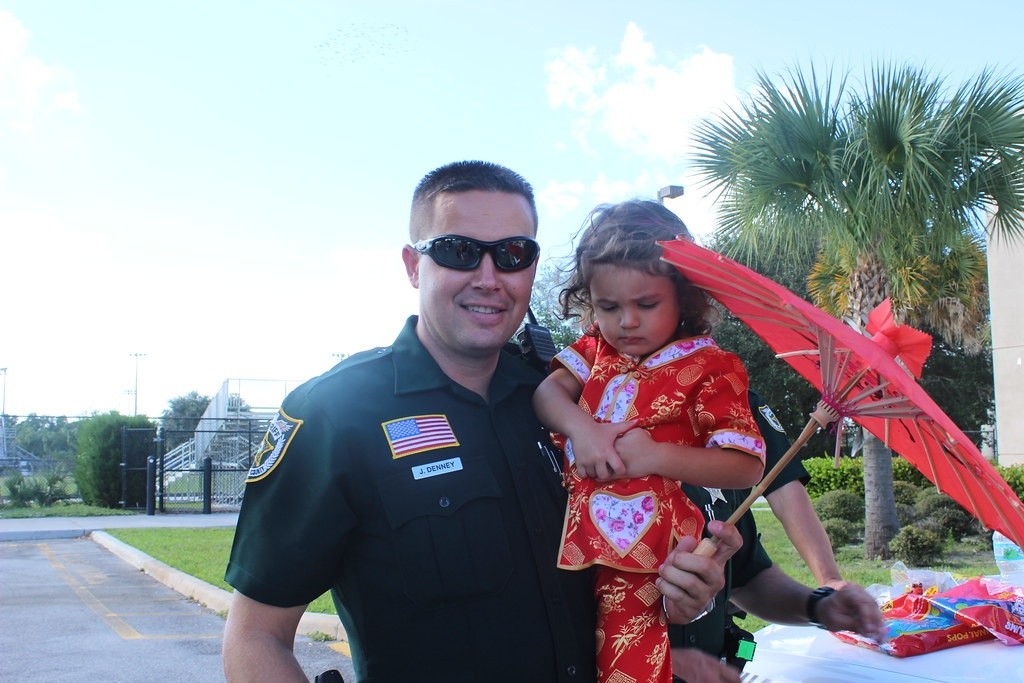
left=224, top=160, right=746, bottom=681
left=662, top=383, right=845, bottom=585
left=532, top=198, right=761, bottom=683
left=654, top=477, right=885, bottom=683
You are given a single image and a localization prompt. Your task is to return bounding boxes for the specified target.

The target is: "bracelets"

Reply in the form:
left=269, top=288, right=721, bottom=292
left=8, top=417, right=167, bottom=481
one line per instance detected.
left=658, top=594, right=715, bottom=624
left=804, top=585, right=841, bottom=629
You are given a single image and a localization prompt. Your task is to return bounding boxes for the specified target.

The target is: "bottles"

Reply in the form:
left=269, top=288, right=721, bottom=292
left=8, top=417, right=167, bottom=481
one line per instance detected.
left=990, top=525, right=1024, bottom=590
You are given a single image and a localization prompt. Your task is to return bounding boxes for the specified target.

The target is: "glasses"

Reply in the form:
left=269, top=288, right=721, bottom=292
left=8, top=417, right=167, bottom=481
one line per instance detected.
left=414, top=234, right=542, bottom=272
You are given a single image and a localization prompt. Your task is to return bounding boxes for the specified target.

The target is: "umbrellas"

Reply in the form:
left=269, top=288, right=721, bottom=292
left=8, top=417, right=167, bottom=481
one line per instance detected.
left=637, top=234, right=1022, bottom=607
